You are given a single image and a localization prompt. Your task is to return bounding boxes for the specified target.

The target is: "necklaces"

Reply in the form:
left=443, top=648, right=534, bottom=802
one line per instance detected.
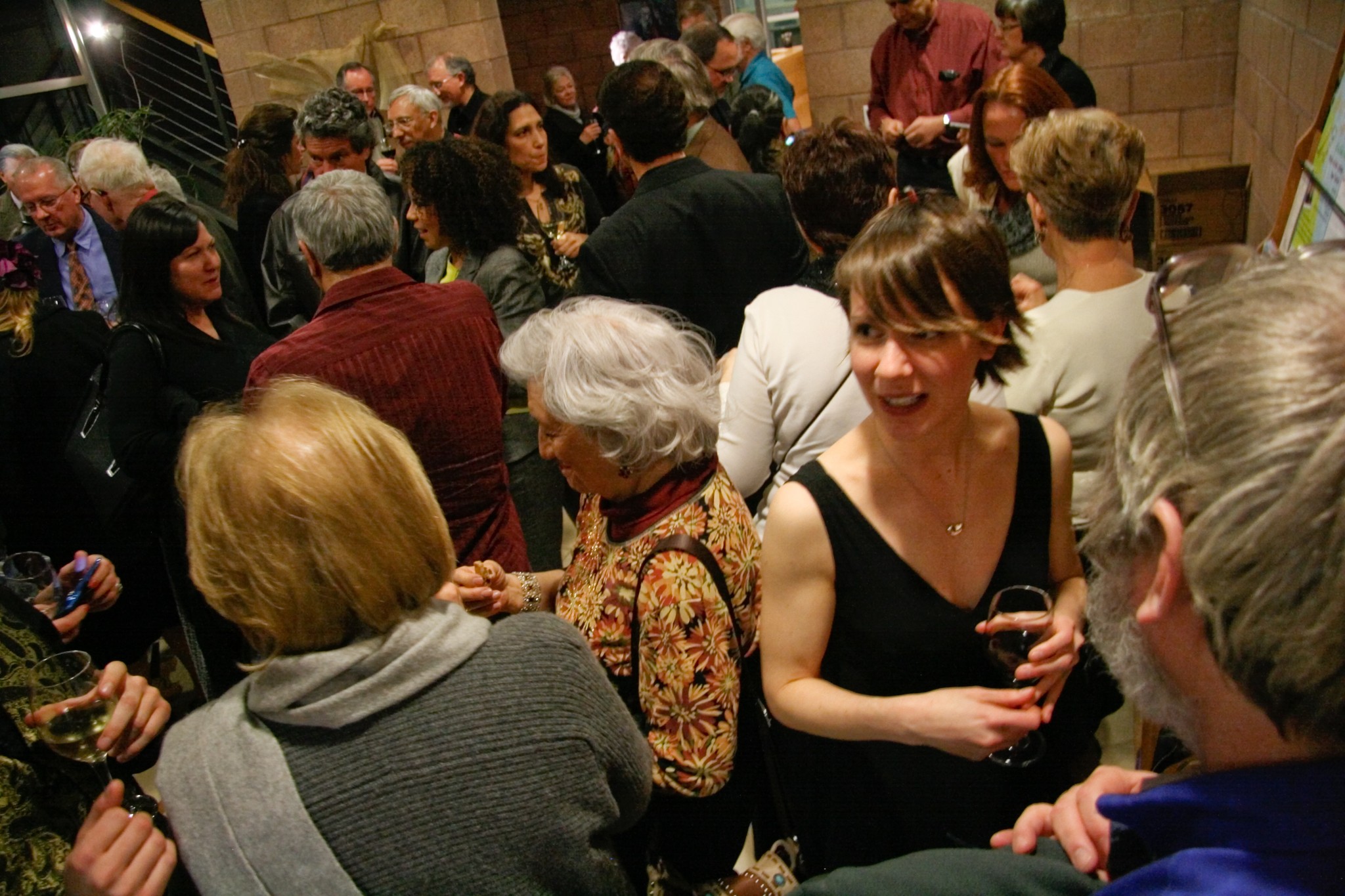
left=875, top=414, right=972, bottom=535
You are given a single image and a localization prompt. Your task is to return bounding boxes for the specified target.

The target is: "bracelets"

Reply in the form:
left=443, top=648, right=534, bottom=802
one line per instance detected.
left=512, top=571, right=542, bottom=612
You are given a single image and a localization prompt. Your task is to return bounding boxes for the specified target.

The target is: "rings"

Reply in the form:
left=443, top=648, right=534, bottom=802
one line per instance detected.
left=116, top=583, right=122, bottom=590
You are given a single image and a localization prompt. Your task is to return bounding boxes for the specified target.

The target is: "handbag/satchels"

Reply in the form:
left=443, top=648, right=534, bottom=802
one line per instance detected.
left=72, top=318, right=177, bottom=496
left=600, top=534, right=774, bottom=875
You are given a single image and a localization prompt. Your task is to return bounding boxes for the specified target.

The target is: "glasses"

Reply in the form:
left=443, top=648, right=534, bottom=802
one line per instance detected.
left=1145, top=235, right=1345, bottom=427
left=20, top=187, right=72, bottom=216
left=436, top=76, right=452, bottom=88
left=383, top=116, right=418, bottom=134
left=705, top=63, right=735, bottom=76
left=81, top=188, right=106, bottom=207
left=1000, top=22, right=1019, bottom=33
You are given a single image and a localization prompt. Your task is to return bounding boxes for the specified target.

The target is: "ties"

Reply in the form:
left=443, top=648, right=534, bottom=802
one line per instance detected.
left=68, top=241, right=94, bottom=312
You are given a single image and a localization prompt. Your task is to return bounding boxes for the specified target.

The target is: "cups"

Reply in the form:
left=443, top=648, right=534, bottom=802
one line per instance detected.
left=379, top=136, right=396, bottom=160
left=0, top=551, right=62, bottom=621
left=42, top=296, right=68, bottom=309
left=92, top=296, right=121, bottom=330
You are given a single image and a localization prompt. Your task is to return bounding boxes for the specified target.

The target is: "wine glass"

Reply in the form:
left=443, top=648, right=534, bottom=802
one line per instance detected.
left=536, top=199, right=575, bottom=273
left=26, top=649, right=160, bottom=819
left=586, top=117, right=603, bottom=155
left=983, top=583, right=1053, bottom=768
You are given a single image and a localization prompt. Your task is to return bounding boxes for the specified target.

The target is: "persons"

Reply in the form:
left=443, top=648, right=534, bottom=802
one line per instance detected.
left=989, top=242, right=1345, bottom=895
left=0, top=0, right=1191, bottom=895
